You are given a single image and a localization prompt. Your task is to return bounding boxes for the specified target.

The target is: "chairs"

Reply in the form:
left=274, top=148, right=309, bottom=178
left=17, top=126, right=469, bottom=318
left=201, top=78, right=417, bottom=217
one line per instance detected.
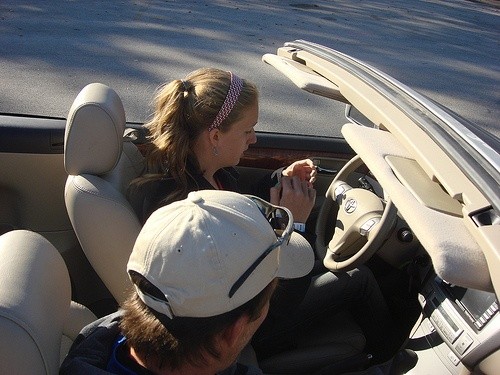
left=62, top=83, right=368, bottom=375
left=1, top=230, right=101, bottom=375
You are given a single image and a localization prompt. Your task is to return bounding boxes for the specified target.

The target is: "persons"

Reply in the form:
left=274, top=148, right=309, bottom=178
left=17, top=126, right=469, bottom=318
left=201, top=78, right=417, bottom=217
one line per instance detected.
left=55, top=189, right=315, bottom=375
left=124, top=65, right=415, bottom=368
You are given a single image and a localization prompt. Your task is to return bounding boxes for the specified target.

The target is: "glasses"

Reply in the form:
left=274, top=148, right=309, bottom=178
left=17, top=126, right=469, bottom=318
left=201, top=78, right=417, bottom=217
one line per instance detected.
left=229, top=190, right=294, bottom=299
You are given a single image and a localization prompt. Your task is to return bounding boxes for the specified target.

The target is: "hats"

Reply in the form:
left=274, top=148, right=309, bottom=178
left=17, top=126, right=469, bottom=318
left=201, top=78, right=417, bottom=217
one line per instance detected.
left=126, top=188, right=315, bottom=318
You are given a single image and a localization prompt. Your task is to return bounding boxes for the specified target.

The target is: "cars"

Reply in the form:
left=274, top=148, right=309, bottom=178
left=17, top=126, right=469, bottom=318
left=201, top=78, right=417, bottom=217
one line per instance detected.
left=0, top=37, right=500, bottom=375
